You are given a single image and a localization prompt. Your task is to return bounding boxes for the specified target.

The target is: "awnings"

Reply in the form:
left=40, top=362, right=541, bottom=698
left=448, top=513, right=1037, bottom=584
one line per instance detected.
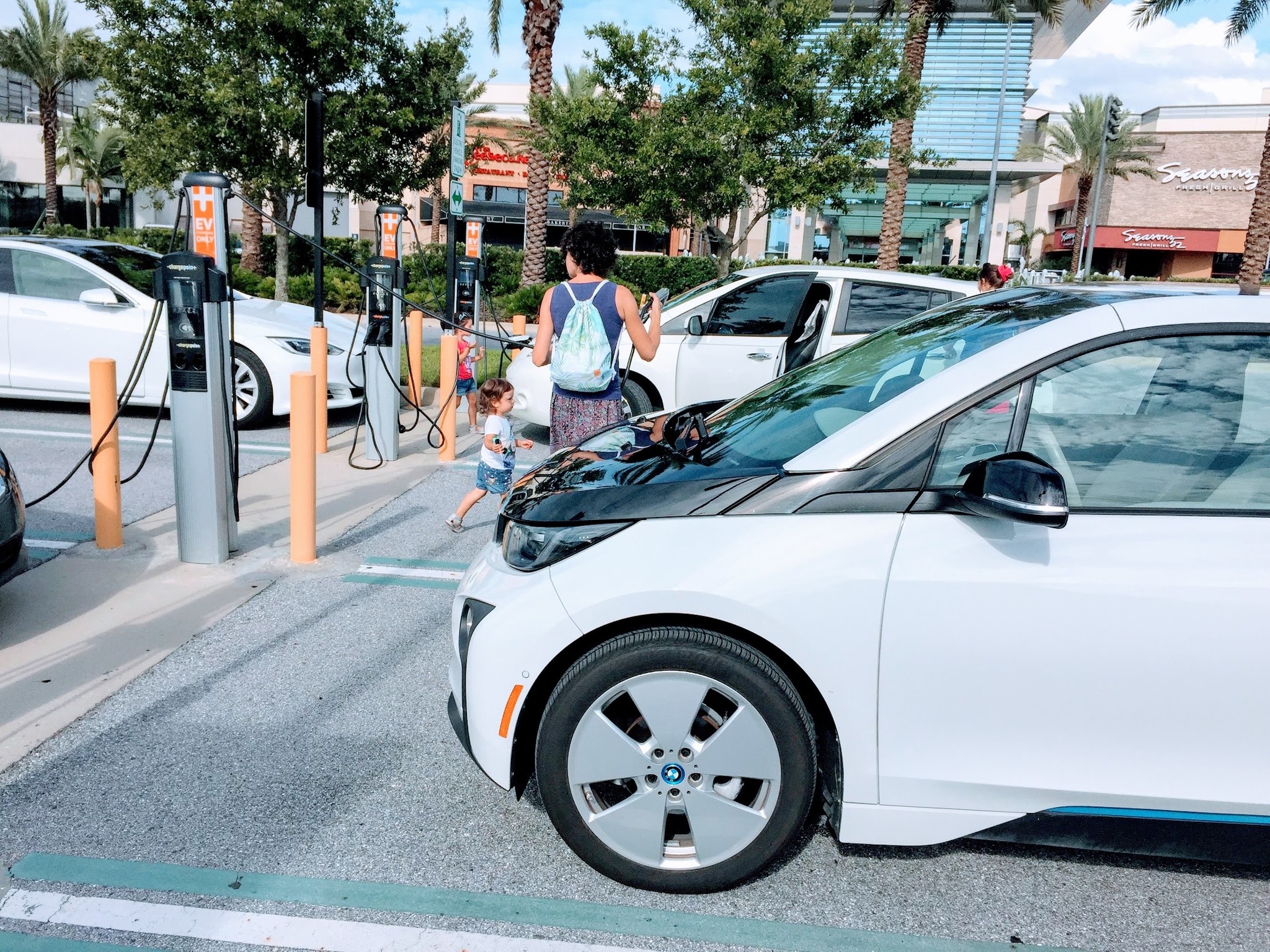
left=420, top=197, right=669, bottom=233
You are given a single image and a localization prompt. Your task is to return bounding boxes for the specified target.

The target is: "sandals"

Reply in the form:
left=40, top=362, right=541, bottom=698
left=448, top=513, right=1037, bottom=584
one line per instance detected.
left=469, top=425, right=485, bottom=434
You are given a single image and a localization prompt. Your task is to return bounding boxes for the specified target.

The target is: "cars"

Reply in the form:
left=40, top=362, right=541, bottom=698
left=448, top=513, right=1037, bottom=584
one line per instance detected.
left=502, top=265, right=994, bottom=452
left=1, top=234, right=369, bottom=429
left=0, top=447, right=28, bottom=573
left=449, top=284, right=1270, bottom=896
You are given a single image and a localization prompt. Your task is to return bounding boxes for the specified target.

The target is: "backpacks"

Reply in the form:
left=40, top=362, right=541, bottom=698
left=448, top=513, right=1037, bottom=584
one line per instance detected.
left=550, top=279, right=623, bottom=392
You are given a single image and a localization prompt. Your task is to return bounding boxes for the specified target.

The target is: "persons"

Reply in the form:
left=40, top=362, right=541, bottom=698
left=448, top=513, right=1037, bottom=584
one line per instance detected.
left=565, top=414, right=672, bottom=463
left=532, top=220, right=663, bottom=455
left=943, top=264, right=1029, bottom=412
left=453, top=311, right=485, bottom=437
left=445, top=378, right=533, bottom=533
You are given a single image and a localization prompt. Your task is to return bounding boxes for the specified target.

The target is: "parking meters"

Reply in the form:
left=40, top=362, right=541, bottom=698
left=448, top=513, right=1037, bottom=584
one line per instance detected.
left=161, top=250, right=240, bottom=565
left=363, top=255, right=408, bottom=460
left=456, top=255, right=480, bottom=412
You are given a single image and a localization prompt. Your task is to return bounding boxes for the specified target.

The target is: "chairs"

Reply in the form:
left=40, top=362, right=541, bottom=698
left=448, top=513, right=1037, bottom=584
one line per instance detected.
left=1081, top=345, right=1270, bottom=505
left=784, top=304, right=826, bottom=374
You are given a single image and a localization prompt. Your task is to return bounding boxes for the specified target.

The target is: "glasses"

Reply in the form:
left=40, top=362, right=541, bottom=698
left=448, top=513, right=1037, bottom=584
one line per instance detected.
left=459, top=311, right=470, bottom=322
left=493, top=436, right=508, bottom=455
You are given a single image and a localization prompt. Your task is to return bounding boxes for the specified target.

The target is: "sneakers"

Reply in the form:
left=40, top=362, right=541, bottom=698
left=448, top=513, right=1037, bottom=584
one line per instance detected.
left=446, top=513, right=465, bottom=533
